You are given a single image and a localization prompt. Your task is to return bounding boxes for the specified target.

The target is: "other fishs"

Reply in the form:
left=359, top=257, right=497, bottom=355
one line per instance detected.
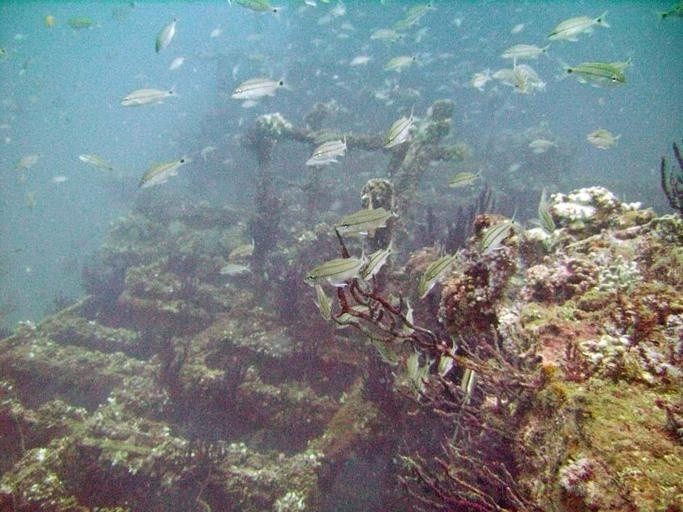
left=1, top=0, right=682, bottom=419
